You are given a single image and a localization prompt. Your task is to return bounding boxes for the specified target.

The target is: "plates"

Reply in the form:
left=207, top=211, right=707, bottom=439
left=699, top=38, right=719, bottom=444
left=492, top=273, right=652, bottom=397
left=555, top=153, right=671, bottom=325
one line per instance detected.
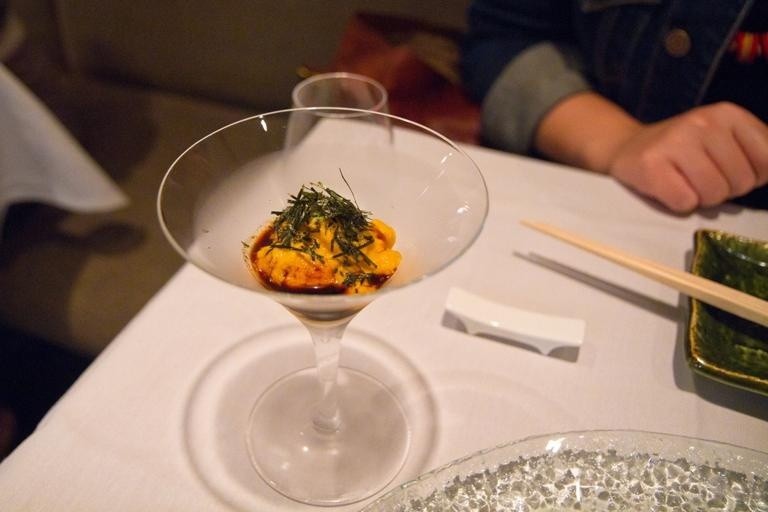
left=684, top=228, right=768, bottom=398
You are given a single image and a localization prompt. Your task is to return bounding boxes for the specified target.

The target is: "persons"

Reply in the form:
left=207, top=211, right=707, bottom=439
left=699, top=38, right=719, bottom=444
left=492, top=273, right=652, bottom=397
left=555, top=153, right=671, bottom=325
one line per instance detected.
left=454, top=0, right=766, bottom=216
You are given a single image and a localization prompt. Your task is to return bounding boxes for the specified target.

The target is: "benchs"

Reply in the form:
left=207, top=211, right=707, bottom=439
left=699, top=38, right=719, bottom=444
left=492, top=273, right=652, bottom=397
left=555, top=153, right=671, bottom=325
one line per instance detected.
left=1, top=2, right=478, bottom=361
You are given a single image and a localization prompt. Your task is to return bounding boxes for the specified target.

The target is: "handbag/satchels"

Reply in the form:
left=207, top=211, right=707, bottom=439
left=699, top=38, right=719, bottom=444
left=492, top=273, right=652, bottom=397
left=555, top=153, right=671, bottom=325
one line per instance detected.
left=293, top=11, right=481, bottom=146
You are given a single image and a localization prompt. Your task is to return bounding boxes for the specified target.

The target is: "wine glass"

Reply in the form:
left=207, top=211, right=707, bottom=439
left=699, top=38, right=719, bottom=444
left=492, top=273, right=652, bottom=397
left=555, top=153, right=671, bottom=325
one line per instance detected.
left=282, top=72, right=394, bottom=180
left=155, top=106, right=489, bottom=507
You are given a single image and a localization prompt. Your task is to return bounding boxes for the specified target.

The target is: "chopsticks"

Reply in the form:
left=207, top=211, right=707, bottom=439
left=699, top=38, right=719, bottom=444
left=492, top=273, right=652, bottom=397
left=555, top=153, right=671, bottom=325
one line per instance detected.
left=520, top=215, right=768, bottom=330
